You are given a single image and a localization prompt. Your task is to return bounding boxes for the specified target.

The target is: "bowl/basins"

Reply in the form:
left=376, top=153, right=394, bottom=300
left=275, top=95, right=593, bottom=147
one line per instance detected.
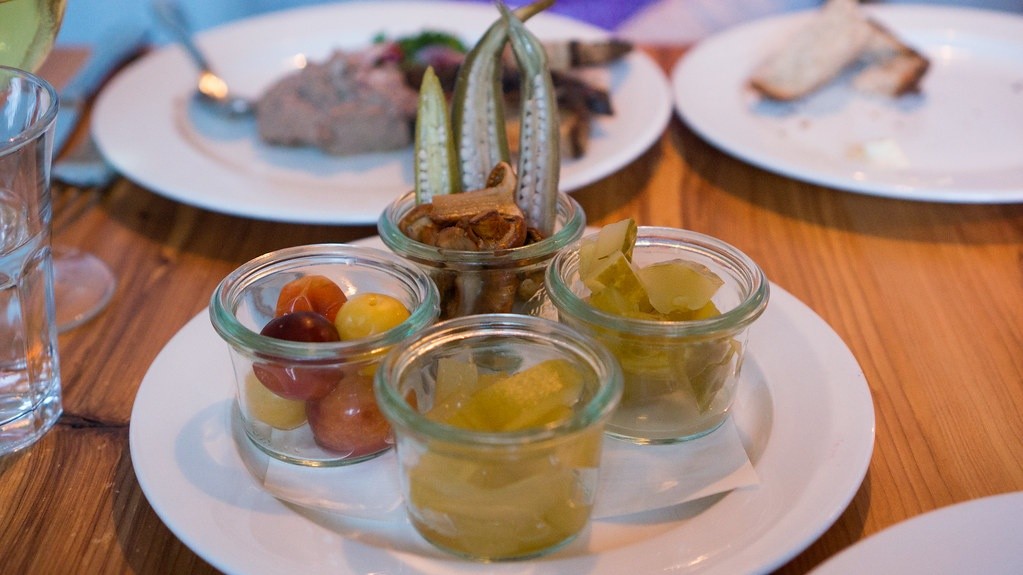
left=373, top=313, right=627, bottom=561
left=544, top=225, right=771, bottom=446
left=377, top=185, right=591, bottom=321
left=206, top=243, right=441, bottom=466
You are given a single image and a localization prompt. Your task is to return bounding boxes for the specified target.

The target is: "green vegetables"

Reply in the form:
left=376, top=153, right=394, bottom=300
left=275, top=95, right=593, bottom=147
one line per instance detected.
left=385, top=29, right=464, bottom=77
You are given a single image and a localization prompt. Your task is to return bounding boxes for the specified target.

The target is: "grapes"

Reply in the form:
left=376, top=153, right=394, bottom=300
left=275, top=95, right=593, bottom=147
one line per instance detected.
left=243, top=275, right=417, bottom=452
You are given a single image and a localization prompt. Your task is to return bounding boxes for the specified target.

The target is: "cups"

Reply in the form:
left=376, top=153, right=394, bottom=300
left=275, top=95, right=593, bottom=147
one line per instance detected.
left=1, top=64, right=64, bottom=460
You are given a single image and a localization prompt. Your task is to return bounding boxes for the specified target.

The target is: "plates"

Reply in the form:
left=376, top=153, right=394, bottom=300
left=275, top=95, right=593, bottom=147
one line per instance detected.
left=91, top=0, right=678, bottom=226
left=802, top=489, right=1023, bottom=575
left=673, top=4, right=1023, bottom=206
left=129, top=230, right=876, bottom=575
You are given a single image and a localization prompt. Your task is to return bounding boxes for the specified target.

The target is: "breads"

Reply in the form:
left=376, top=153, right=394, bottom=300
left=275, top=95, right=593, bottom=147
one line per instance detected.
left=752, top=0, right=931, bottom=102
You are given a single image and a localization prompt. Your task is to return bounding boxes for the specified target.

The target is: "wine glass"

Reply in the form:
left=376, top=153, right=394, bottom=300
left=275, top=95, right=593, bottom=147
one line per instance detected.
left=43, top=241, right=116, bottom=332
